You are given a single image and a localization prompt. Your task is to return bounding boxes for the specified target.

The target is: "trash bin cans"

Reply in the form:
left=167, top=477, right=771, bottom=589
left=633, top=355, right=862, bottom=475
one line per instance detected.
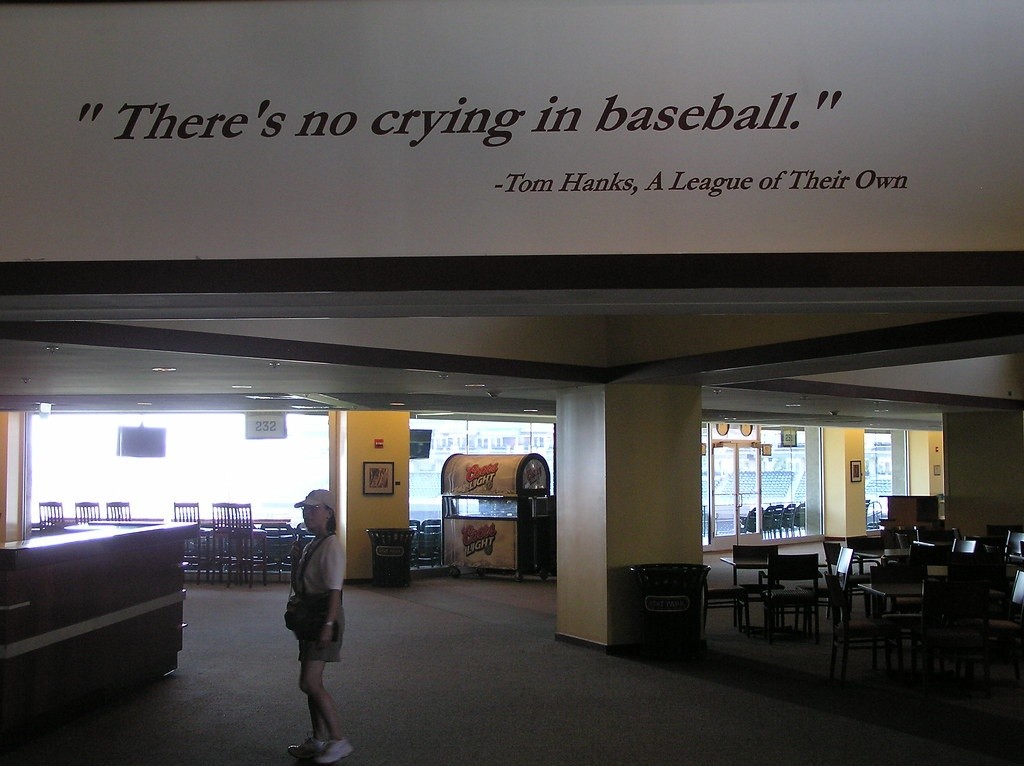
left=365, top=528, right=415, bottom=587
left=629, top=564, right=712, bottom=663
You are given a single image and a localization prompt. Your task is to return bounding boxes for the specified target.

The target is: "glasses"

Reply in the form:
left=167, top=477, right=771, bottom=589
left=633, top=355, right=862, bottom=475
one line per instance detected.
left=301, top=505, right=321, bottom=511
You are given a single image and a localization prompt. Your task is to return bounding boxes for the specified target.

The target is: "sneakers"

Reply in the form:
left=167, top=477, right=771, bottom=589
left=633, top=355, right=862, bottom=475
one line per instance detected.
left=287, top=737, right=330, bottom=759
left=314, top=736, right=354, bottom=764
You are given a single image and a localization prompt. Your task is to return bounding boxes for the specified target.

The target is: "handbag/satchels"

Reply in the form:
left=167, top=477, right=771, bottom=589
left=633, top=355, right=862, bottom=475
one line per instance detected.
left=284, top=589, right=339, bottom=642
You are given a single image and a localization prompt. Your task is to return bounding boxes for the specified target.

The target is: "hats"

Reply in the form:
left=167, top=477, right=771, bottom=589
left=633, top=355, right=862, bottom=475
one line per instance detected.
left=294, top=488, right=336, bottom=512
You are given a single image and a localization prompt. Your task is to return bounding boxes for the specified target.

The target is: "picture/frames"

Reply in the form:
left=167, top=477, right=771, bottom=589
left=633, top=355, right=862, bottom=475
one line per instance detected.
left=362, top=462, right=395, bottom=494
left=850, top=460, right=861, bottom=482
left=934, top=465, right=940, bottom=476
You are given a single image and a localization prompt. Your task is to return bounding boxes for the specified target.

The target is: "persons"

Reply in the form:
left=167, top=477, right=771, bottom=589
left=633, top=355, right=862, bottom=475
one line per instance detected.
left=284, top=489, right=355, bottom=764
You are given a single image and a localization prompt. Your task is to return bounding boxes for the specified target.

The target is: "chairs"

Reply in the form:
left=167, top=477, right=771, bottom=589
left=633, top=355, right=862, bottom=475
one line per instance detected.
left=39, top=502, right=442, bottom=588
left=705, top=500, right=1024, bottom=700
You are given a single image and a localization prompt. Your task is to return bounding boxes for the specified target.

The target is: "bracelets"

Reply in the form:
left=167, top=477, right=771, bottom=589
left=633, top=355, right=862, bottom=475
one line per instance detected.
left=325, top=622, right=336, bottom=626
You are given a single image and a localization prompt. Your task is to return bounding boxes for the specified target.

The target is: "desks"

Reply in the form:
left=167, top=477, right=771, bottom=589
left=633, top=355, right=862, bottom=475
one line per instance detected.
left=856, top=581, right=1006, bottom=671
left=171, top=517, right=291, bottom=583
left=720, top=557, right=827, bottom=641
